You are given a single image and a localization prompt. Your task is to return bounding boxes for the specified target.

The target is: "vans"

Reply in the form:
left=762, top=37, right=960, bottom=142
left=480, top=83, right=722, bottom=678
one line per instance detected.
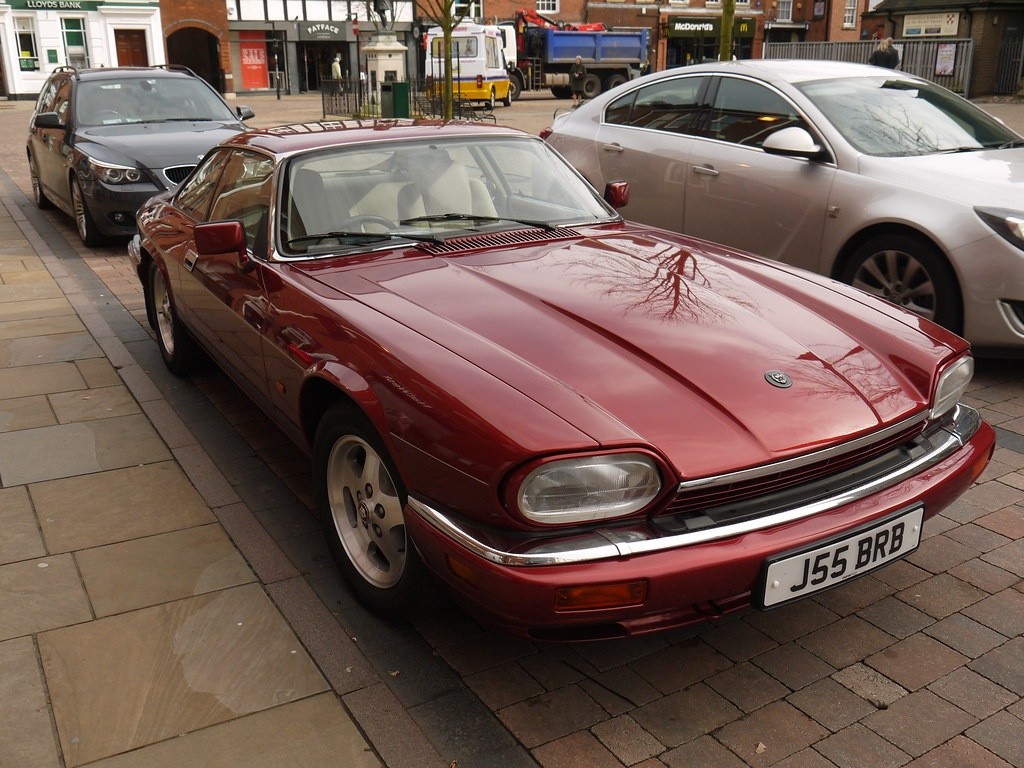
left=425, top=22, right=513, bottom=111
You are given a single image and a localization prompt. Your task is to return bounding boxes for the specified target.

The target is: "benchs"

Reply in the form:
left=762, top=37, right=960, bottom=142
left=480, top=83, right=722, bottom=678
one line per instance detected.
left=413, top=92, right=497, bottom=124
left=322, top=168, right=413, bottom=233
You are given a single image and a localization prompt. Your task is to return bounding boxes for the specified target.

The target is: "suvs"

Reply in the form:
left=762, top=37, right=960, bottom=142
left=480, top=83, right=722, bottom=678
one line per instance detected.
left=25, top=64, right=259, bottom=249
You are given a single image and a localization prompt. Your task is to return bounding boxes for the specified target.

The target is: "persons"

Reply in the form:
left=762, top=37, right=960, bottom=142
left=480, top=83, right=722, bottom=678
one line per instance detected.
left=330, top=57, right=343, bottom=96
left=641, top=60, right=652, bottom=76
left=867, top=37, right=899, bottom=70
left=571, top=55, right=587, bottom=108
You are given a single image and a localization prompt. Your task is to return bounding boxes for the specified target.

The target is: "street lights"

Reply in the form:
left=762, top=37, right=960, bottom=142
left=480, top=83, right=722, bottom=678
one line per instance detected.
left=265, top=20, right=282, bottom=100
left=346, top=12, right=363, bottom=107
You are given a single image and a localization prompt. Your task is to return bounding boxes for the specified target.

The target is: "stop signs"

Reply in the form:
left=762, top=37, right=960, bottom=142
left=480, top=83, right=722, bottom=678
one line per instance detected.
left=351, top=18, right=360, bottom=36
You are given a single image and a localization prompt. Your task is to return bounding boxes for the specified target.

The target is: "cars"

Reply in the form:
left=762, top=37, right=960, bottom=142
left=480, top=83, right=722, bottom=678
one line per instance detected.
left=526, top=57, right=1024, bottom=346
left=123, top=113, right=997, bottom=656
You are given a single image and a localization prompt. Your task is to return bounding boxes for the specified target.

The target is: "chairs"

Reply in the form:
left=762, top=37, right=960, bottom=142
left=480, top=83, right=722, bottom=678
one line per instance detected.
left=252, top=169, right=354, bottom=261
left=398, top=155, right=499, bottom=231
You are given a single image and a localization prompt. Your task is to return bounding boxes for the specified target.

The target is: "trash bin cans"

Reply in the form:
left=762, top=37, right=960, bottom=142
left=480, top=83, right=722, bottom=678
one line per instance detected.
left=380, top=81, right=411, bottom=118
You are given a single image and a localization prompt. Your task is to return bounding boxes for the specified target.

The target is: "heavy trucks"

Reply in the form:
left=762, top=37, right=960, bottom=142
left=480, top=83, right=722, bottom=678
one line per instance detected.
left=489, top=25, right=649, bottom=103
